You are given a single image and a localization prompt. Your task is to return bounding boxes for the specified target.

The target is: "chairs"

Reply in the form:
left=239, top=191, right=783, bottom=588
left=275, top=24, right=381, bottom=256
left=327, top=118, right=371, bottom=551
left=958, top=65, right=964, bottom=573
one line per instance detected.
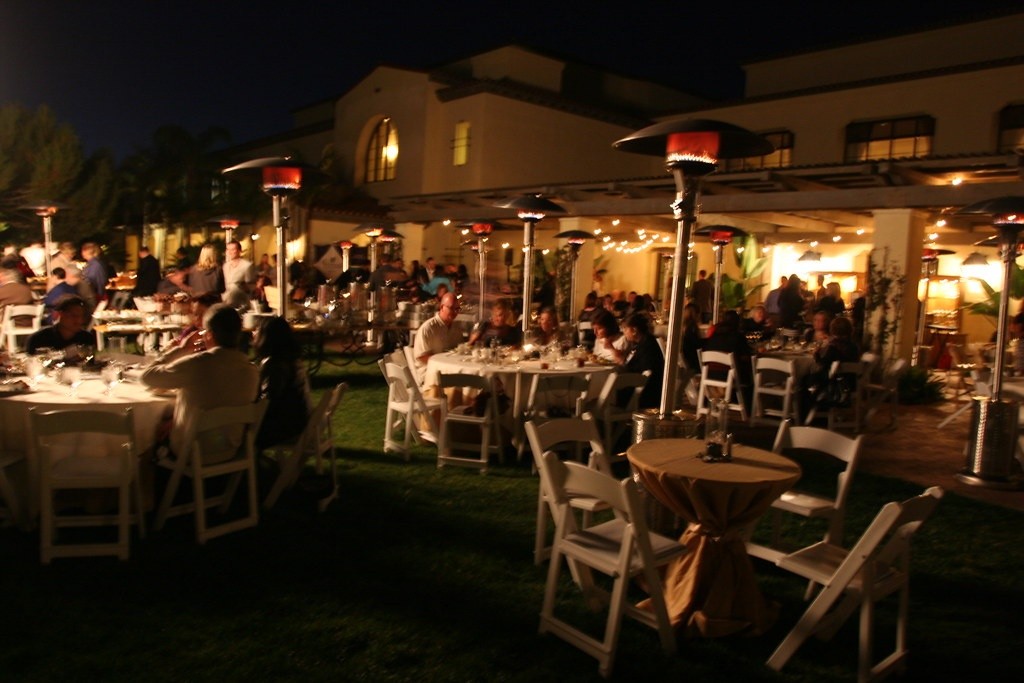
left=0, top=298, right=1024, bottom=683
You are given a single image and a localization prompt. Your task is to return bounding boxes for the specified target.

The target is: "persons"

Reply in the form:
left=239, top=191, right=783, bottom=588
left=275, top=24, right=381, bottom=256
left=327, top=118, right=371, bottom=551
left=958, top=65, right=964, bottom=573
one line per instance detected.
left=720, top=273, right=740, bottom=307
left=414, top=292, right=477, bottom=405
left=591, top=309, right=629, bottom=365
left=532, top=308, right=574, bottom=351
left=852, top=289, right=865, bottom=343
left=691, top=270, right=714, bottom=323
left=255, top=317, right=309, bottom=476
left=617, top=309, right=665, bottom=413
left=819, top=282, right=845, bottom=332
left=805, top=311, right=829, bottom=341
left=474, top=297, right=521, bottom=416
left=578, top=292, right=603, bottom=353
left=370, top=253, right=470, bottom=304
left=799, top=316, right=858, bottom=428
left=679, top=318, right=702, bottom=374
left=151, top=303, right=258, bottom=499
left=0, top=240, right=309, bottom=356
left=604, top=291, right=656, bottom=320
left=706, top=310, right=762, bottom=415
left=736, top=274, right=826, bottom=340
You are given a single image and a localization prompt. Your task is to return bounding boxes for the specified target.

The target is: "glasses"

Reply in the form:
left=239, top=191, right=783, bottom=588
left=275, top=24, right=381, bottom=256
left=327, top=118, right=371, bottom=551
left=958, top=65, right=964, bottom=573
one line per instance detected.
left=442, top=305, right=461, bottom=313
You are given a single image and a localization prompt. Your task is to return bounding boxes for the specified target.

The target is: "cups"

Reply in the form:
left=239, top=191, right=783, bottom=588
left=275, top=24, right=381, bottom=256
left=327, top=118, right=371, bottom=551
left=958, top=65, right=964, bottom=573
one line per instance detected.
left=496, top=357, right=504, bottom=369
left=540, top=352, right=549, bottom=369
left=706, top=398, right=728, bottom=460
left=54, top=367, right=82, bottom=398
left=193, top=332, right=205, bottom=350
left=573, top=352, right=584, bottom=367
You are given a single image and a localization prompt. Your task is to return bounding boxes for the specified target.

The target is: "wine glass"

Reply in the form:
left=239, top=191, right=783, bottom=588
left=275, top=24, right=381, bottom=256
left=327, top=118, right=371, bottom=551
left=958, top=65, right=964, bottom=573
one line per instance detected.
left=510, top=349, right=522, bottom=370
left=101, top=359, right=128, bottom=397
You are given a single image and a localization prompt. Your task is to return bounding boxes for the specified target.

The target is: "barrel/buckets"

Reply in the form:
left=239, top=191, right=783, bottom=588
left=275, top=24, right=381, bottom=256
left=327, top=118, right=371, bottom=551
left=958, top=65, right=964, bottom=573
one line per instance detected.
left=348, top=282, right=370, bottom=324
left=316, top=284, right=337, bottom=307
left=373, top=285, right=396, bottom=323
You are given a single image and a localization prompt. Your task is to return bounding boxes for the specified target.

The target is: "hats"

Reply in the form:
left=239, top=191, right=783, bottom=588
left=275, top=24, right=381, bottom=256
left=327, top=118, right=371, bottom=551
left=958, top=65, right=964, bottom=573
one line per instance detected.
left=49, top=294, right=88, bottom=312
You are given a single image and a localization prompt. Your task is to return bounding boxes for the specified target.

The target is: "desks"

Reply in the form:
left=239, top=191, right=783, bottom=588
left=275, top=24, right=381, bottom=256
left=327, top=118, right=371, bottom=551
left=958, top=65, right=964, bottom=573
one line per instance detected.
left=627, top=439, right=801, bottom=639
left=282, top=303, right=421, bottom=374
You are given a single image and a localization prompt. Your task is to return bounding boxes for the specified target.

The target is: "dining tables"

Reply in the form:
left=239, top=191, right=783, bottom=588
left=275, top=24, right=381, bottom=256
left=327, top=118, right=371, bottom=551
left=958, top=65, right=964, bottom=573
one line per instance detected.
left=426, top=349, right=622, bottom=452
left=749, top=340, right=818, bottom=389
left=1, top=373, right=179, bottom=483
